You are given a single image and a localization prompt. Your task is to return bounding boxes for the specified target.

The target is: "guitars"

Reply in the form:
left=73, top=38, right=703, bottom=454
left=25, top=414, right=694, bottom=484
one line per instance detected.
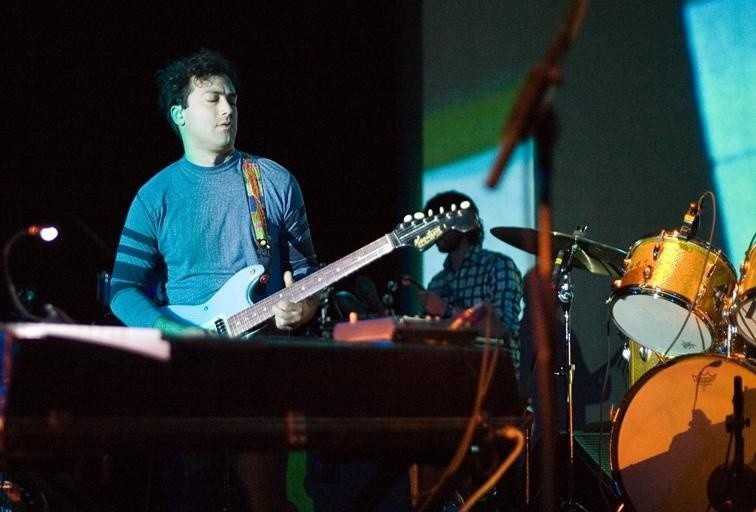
left=158, top=201, right=483, bottom=341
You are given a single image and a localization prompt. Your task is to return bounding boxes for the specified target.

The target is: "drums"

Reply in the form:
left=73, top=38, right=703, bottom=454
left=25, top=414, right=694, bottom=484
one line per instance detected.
left=721, top=234, right=756, bottom=346
left=608, top=354, right=756, bottom=512
left=604, top=230, right=737, bottom=356
left=622, top=338, right=675, bottom=389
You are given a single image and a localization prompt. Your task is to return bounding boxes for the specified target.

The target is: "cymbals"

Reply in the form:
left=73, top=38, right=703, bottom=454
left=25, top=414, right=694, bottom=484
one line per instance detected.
left=490, top=227, right=627, bottom=277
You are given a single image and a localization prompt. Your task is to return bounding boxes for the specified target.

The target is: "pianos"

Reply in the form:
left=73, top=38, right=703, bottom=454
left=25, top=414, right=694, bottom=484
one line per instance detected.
left=333, top=317, right=453, bottom=342
left=1, top=330, right=529, bottom=447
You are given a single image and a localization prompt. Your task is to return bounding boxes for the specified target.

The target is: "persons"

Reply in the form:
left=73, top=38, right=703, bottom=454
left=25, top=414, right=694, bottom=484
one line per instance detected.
left=417, top=191, right=521, bottom=394
left=108, top=47, right=321, bottom=342
